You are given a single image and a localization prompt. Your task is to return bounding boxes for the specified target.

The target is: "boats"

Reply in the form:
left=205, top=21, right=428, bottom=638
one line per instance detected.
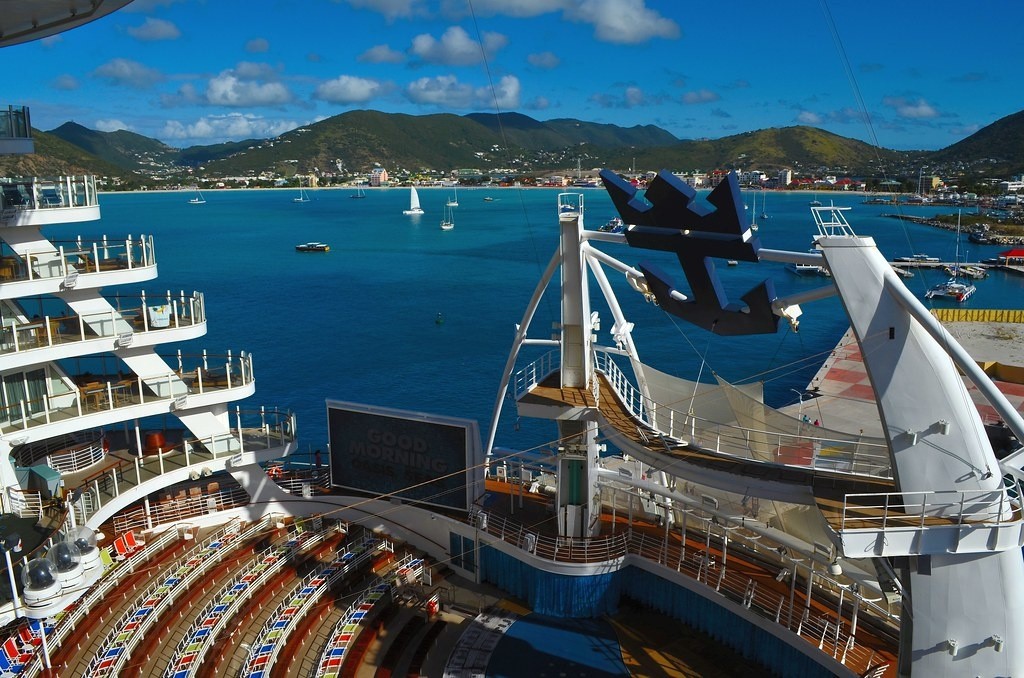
left=187, top=184, right=207, bottom=204
left=893, top=249, right=940, bottom=269
left=810, top=192, right=822, bottom=206
left=295, top=241, right=331, bottom=251
left=968, top=230, right=988, bottom=246
left=942, top=246, right=989, bottom=280
left=923, top=208, right=977, bottom=302
left=786, top=232, right=832, bottom=276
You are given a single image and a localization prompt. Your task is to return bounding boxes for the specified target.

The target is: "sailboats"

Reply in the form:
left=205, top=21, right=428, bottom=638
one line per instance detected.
left=402, top=185, right=425, bottom=216
left=446, top=186, right=459, bottom=206
left=291, top=178, right=312, bottom=202
left=350, top=178, right=367, bottom=198
left=750, top=189, right=758, bottom=231
left=761, top=188, right=768, bottom=219
left=558, top=187, right=576, bottom=212
left=440, top=196, right=456, bottom=231
